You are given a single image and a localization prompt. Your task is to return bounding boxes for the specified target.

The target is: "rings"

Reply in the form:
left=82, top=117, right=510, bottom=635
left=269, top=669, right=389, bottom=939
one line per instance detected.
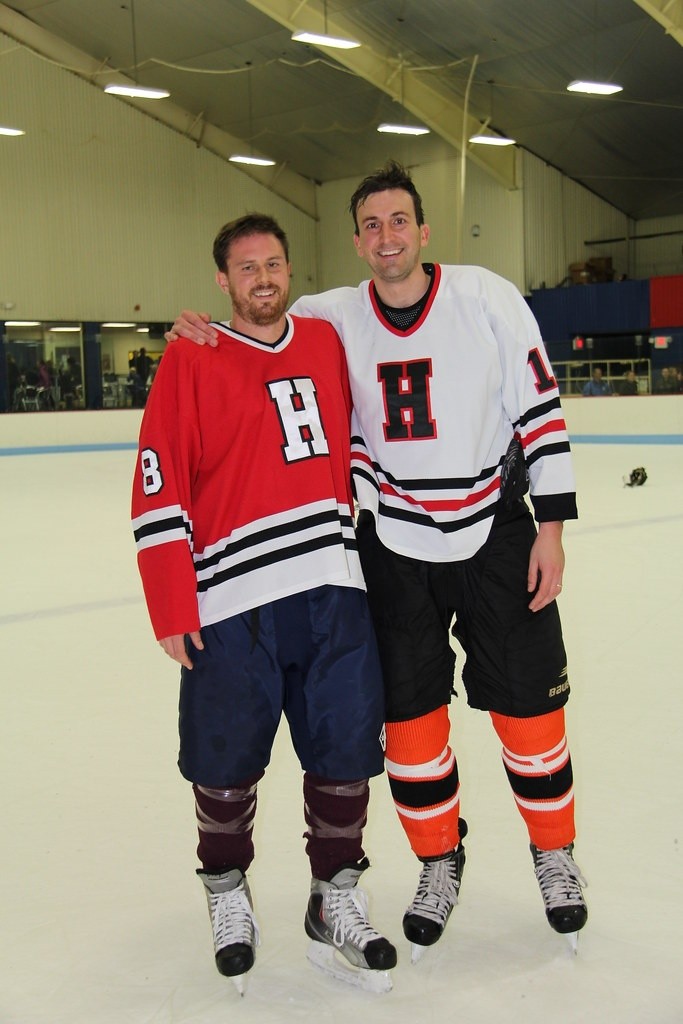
left=555, top=583, right=563, bottom=589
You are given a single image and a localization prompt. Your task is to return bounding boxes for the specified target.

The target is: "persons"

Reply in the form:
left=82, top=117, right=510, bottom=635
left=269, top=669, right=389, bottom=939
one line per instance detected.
left=162, top=156, right=590, bottom=950
left=581, top=367, right=611, bottom=396
left=126, top=212, right=415, bottom=943
left=652, top=365, right=682, bottom=396
left=618, top=370, right=638, bottom=398
left=3, top=317, right=166, bottom=415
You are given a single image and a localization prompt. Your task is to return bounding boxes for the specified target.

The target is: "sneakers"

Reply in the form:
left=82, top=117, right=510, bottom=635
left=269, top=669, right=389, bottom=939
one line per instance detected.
left=401, top=817, right=468, bottom=966
left=195, top=865, right=262, bottom=997
left=303, top=857, right=397, bottom=994
left=529, top=841, right=588, bottom=949
